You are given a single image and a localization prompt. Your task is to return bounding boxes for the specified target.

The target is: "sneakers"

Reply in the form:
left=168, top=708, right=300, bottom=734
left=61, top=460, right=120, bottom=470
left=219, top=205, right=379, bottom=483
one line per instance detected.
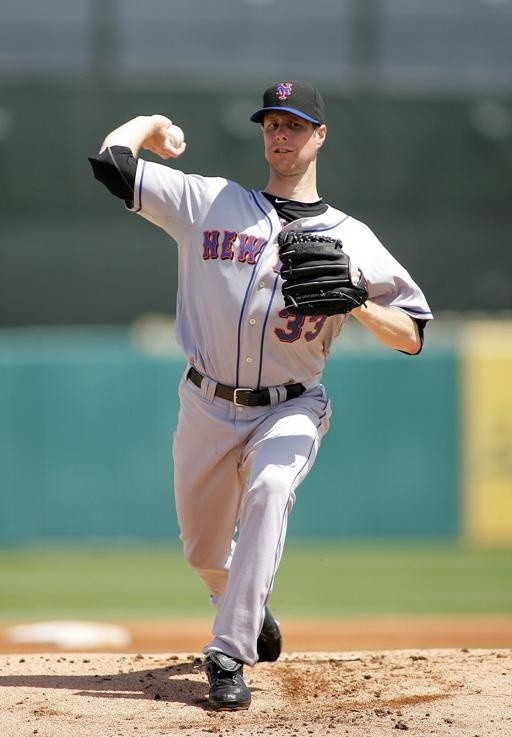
left=257, top=606, right=280, bottom=660
left=204, top=651, right=251, bottom=710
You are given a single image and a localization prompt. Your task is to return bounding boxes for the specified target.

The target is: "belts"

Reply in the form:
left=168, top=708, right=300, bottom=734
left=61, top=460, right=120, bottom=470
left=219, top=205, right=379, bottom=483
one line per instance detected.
left=188, top=367, right=304, bottom=406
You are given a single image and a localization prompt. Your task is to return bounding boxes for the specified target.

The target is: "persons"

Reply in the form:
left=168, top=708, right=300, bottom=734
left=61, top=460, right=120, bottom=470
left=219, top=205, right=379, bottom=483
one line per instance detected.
left=90, top=78, right=435, bottom=710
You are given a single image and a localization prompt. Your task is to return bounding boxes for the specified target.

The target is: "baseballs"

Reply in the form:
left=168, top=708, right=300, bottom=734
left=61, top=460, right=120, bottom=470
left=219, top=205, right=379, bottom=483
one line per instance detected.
left=170, top=125, right=184, bottom=148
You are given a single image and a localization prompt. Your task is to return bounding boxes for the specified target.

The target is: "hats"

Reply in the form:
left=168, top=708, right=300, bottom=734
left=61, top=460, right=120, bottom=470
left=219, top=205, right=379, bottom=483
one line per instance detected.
left=251, top=82, right=325, bottom=126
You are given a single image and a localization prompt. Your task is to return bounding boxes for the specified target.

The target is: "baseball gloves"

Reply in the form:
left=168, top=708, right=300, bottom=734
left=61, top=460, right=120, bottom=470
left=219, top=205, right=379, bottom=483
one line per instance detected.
left=277, top=230, right=367, bottom=316
